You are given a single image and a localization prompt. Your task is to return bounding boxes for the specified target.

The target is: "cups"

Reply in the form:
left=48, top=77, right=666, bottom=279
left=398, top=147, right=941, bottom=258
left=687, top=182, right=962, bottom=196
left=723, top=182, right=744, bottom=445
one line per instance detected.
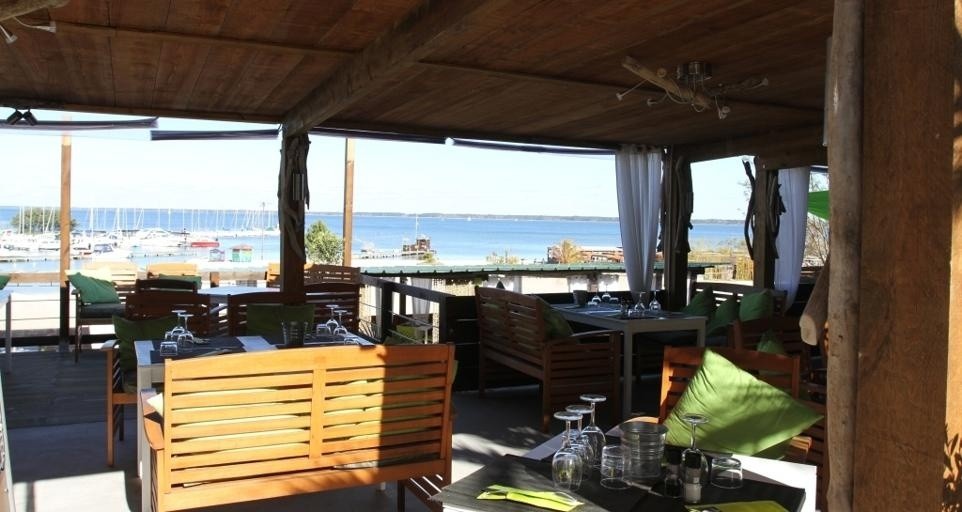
left=711, top=457, right=743, bottom=490
left=344, top=336, right=358, bottom=345
left=164, top=331, right=170, bottom=341
left=609, top=298, right=619, bottom=309
left=628, top=308, right=646, bottom=318
left=588, top=303, right=597, bottom=311
left=316, top=324, right=328, bottom=336
left=600, top=444, right=633, bottom=490
left=160, top=342, right=178, bottom=357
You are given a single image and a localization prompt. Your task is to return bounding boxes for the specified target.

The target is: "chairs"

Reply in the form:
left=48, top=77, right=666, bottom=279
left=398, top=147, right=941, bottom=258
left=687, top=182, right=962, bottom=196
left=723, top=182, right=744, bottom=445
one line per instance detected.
left=729, top=320, right=826, bottom=454
left=607, top=341, right=814, bottom=464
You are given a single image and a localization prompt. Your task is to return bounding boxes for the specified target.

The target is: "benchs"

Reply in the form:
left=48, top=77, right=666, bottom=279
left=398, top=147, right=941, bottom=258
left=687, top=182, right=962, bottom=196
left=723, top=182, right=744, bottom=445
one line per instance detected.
left=140, top=344, right=458, bottom=511
left=685, top=280, right=790, bottom=348
left=474, top=283, right=621, bottom=434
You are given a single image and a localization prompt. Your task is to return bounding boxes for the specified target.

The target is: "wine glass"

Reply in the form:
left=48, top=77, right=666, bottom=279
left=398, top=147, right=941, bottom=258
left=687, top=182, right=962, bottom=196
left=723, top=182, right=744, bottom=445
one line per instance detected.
left=177, top=314, right=194, bottom=353
left=680, top=414, right=709, bottom=482
left=552, top=411, right=583, bottom=492
left=649, top=289, right=661, bottom=313
left=171, top=310, right=187, bottom=341
left=565, top=404, right=593, bottom=482
left=634, top=291, right=646, bottom=311
left=326, top=305, right=339, bottom=335
left=592, top=285, right=601, bottom=308
left=602, top=282, right=611, bottom=308
left=333, top=309, right=347, bottom=341
left=580, top=394, right=607, bottom=467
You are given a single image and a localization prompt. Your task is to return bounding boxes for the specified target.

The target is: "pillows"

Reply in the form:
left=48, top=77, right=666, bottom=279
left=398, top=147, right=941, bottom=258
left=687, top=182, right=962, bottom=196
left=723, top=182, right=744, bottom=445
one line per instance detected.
left=658, top=348, right=824, bottom=461
left=0, top=274, right=12, bottom=290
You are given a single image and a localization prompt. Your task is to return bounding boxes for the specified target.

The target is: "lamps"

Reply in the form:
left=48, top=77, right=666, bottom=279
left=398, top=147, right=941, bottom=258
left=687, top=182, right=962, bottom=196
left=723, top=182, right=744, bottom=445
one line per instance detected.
left=7, top=108, right=38, bottom=126
left=678, top=59, right=713, bottom=82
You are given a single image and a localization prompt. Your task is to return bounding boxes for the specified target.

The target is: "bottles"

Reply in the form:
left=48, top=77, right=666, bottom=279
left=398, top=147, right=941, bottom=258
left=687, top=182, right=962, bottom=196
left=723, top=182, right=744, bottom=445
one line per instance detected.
left=683, top=477, right=702, bottom=504
left=621, top=299, right=631, bottom=312
left=665, top=473, right=683, bottom=498
